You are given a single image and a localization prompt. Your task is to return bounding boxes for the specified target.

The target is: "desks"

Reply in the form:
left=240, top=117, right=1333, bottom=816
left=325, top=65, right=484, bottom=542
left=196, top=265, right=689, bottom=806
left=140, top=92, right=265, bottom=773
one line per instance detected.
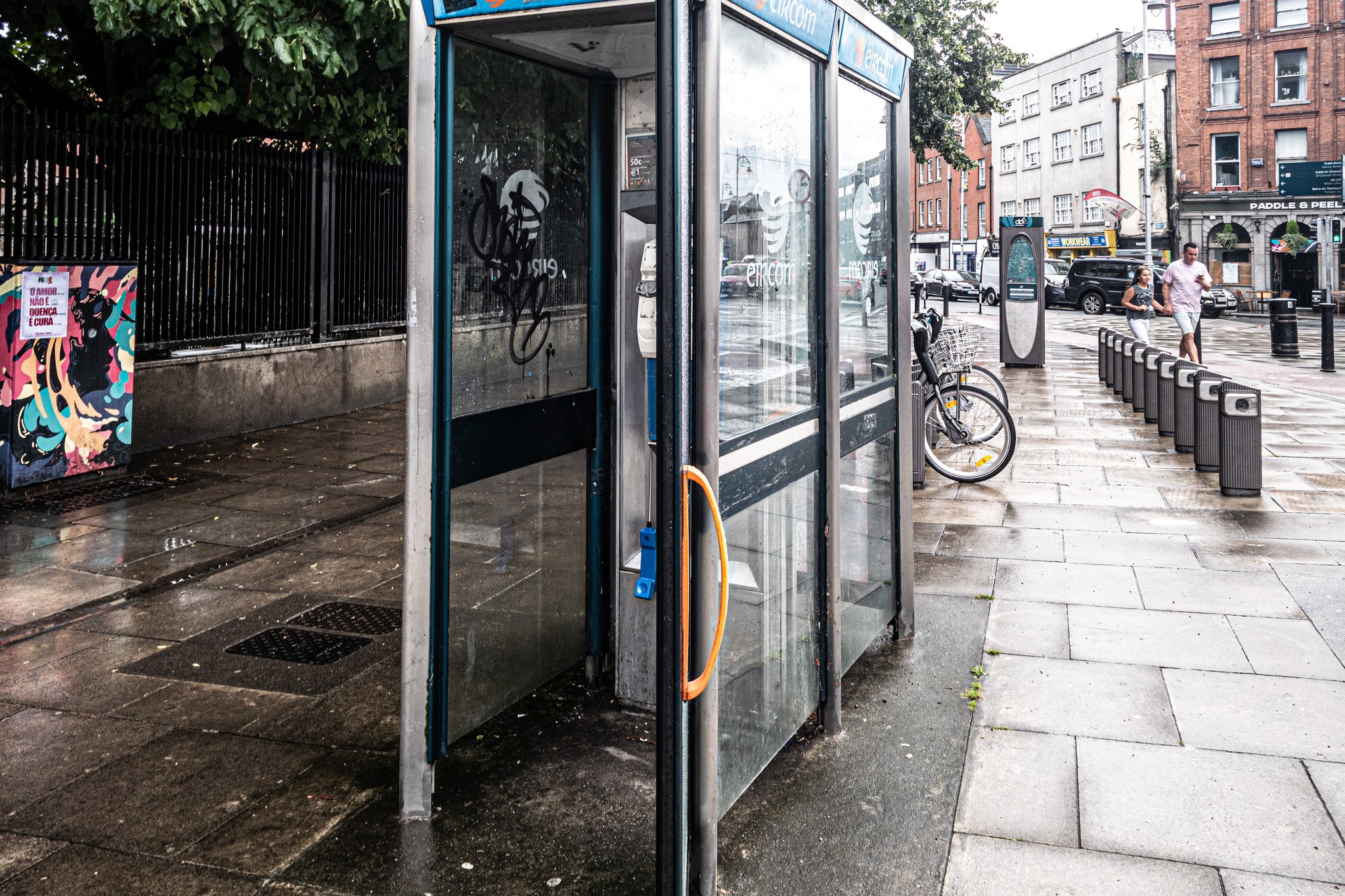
left=1245, top=291, right=1279, bottom=314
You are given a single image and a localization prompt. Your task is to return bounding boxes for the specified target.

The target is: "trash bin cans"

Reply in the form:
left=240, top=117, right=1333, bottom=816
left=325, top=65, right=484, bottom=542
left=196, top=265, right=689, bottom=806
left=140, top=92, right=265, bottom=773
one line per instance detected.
left=1268, top=297, right=1301, bottom=357
left=1311, top=289, right=1326, bottom=313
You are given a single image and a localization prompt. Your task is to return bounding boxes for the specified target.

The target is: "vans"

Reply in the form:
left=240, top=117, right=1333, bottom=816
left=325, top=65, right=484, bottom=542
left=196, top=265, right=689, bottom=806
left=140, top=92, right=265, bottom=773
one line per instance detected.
left=980, top=257, right=1076, bottom=310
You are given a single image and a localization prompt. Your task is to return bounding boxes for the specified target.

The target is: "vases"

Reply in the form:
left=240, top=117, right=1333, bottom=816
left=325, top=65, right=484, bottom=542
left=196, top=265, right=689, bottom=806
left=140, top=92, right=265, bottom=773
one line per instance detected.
left=1214, top=186, right=1239, bottom=191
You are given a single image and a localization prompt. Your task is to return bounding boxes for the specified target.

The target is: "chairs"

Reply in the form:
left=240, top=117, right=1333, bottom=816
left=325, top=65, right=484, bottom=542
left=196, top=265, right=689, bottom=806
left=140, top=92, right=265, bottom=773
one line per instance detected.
left=1257, top=290, right=1271, bottom=314
left=1332, top=291, right=1345, bottom=315
left=1281, top=290, right=1291, bottom=298
left=1236, top=289, right=1256, bottom=314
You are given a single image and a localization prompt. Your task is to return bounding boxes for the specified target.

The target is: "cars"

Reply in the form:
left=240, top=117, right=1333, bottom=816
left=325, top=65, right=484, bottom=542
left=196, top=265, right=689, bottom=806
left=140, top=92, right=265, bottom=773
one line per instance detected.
left=920, top=268, right=987, bottom=303
left=720, top=254, right=805, bottom=299
left=838, top=266, right=876, bottom=307
left=879, top=267, right=887, bottom=286
left=910, top=271, right=928, bottom=297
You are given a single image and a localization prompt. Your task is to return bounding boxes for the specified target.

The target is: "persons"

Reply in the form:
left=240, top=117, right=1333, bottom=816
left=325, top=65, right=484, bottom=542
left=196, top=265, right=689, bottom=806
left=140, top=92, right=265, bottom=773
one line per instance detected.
left=916, top=265, right=924, bottom=271
left=913, top=263, right=920, bottom=271
left=879, top=264, right=882, bottom=269
left=1161, top=242, right=1211, bottom=365
left=722, top=258, right=747, bottom=275
left=1122, top=266, right=1173, bottom=346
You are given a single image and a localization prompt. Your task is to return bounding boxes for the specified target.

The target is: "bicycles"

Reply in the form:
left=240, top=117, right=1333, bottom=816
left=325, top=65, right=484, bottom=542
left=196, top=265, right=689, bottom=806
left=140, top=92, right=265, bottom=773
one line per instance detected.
left=910, top=307, right=1019, bottom=485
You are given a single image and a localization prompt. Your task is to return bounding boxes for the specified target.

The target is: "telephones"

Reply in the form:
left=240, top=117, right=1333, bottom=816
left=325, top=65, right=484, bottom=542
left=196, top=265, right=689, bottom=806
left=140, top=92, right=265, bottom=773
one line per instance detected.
left=634, top=527, right=656, bottom=600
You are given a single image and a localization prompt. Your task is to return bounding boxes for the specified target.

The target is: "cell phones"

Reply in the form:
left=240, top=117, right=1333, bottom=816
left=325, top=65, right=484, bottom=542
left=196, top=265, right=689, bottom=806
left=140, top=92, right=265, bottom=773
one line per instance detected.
left=1196, top=275, right=1204, bottom=281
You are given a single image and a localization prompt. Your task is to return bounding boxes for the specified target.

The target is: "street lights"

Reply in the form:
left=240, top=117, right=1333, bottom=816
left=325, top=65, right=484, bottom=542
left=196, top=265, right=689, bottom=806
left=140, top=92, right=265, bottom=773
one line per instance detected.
left=1140, top=0, right=1172, bottom=318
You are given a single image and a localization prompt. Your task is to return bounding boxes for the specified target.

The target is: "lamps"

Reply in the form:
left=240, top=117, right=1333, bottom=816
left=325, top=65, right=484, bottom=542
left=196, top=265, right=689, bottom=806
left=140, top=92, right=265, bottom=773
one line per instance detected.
left=1219, top=197, right=1229, bottom=201
left=1254, top=218, right=1261, bottom=233
left=1284, top=195, right=1293, bottom=199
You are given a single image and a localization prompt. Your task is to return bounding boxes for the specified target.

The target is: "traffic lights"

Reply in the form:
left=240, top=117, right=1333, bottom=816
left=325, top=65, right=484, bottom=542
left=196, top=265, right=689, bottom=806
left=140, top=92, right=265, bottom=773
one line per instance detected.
left=1331, top=219, right=1342, bottom=244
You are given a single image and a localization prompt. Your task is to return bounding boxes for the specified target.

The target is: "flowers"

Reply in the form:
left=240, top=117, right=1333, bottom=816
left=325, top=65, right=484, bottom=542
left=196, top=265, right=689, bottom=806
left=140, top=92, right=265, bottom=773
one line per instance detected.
left=1229, top=183, right=1231, bottom=186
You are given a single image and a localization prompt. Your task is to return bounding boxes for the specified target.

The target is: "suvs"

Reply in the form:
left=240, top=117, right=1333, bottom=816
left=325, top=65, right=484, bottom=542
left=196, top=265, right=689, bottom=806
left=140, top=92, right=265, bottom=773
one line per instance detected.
left=1062, top=256, right=1238, bottom=319
